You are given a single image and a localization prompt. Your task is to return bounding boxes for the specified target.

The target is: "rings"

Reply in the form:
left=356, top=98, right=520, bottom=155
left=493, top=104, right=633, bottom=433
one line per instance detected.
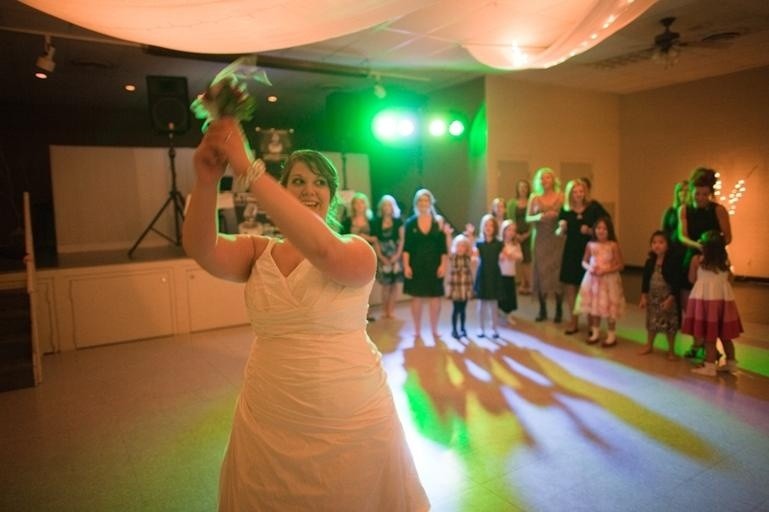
left=225, top=132, right=233, bottom=144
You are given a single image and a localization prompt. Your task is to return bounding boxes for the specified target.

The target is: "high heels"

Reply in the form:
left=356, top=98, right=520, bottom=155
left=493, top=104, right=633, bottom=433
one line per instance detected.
left=685, top=342, right=705, bottom=360
left=715, top=348, right=724, bottom=364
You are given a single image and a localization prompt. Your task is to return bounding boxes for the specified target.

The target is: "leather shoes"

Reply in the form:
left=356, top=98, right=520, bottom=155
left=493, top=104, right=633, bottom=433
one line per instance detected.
left=602, top=338, right=618, bottom=347
left=584, top=335, right=598, bottom=344
left=566, top=328, right=579, bottom=336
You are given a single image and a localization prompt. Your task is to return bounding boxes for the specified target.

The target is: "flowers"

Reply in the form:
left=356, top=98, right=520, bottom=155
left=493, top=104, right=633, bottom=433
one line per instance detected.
left=188, top=54, right=273, bottom=136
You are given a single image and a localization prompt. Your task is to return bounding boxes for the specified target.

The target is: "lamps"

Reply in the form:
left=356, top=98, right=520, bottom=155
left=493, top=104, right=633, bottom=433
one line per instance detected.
left=33, top=35, right=56, bottom=80
left=649, top=18, right=682, bottom=71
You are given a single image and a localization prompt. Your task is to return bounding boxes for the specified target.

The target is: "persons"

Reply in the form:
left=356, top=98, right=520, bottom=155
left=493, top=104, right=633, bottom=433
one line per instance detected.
left=677, top=167, right=732, bottom=363
left=506, top=179, right=534, bottom=295
left=488, top=197, right=505, bottom=226
left=497, top=220, right=524, bottom=326
left=639, top=231, right=683, bottom=363
left=444, top=234, right=470, bottom=339
left=553, top=178, right=617, bottom=339
left=572, top=216, right=626, bottom=349
left=680, top=232, right=743, bottom=377
left=181, top=118, right=431, bottom=512
left=466, top=214, right=504, bottom=339
left=400, top=188, right=449, bottom=342
left=524, top=166, right=570, bottom=324
left=661, top=180, right=689, bottom=330
left=369, top=194, right=404, bottom=321
left=340, top=192, right=376, bottom=322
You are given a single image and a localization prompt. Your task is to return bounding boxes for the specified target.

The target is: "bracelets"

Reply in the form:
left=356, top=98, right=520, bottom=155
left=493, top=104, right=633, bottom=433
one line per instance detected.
left=230, top=158, right=268, bottom=196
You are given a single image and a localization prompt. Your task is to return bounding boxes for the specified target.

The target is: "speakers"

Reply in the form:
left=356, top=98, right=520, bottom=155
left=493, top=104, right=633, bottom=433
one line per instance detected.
left=145, top=74, right=192, bottom=137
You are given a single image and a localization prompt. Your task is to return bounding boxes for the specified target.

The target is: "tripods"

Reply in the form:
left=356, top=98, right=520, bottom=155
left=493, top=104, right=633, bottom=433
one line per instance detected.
left=127, top=138, right=190, bottom=255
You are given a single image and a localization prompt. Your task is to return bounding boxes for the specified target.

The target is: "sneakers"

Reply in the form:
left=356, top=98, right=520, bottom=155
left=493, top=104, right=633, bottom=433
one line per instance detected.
left=718, top=359, right=738, bottom=372
left=692, top=362, right=716, bottom=378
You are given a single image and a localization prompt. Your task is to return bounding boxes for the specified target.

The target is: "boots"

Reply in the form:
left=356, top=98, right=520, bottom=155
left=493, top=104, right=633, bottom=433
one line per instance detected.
left=554, top=293, right=564, bottom=323
left=536, top=293, right=548, bottom=321
